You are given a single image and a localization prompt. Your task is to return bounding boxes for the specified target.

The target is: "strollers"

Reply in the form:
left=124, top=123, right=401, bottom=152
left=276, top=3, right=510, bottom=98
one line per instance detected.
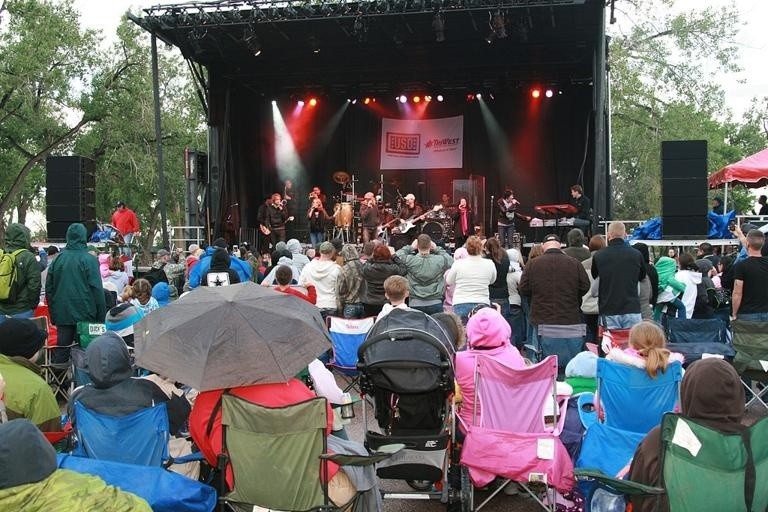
left=357, top=311, right=477, bottom=507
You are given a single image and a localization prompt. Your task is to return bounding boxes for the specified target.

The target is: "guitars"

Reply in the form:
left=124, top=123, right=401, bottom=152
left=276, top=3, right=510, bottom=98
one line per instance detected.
left=398, top=204, right=442, bottom=234
left=373, top=214, right=400, bottom=239
left=259, top=216, right=294, bottom=235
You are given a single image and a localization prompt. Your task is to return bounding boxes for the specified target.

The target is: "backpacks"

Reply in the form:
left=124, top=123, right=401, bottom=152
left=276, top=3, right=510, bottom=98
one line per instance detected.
left=0, top=249, right=28, bottom=299
left=703, top=279, right=732, bottom=308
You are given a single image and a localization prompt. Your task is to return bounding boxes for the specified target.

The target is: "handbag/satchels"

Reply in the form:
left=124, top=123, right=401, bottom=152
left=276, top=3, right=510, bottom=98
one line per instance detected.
left=166, top=433, right=200, bottom=482
left=76, top=322, right=107, bottom=349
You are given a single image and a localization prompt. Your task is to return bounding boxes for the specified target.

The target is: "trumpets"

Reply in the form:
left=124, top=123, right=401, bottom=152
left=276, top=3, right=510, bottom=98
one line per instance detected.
left=306, top=191, right=317, bottom=200
left=269, top=197, right=288, bottom=210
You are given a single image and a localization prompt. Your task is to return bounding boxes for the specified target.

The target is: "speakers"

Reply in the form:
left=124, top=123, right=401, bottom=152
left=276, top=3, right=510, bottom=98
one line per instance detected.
left=662, top=140, right=707, bottom=240
left=46, top=156, right=96, bottom=242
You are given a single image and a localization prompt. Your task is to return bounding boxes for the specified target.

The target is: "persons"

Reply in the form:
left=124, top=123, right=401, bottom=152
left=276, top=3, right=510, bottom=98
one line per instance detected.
left=0, top=170, right=768, bottom=510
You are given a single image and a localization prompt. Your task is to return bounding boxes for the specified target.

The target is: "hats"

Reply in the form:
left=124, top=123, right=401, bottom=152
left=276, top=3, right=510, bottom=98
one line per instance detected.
left=0, top=319, right=48, bottom=359
left=319, top=242, right=333, bottom=253
left=364, top=192, right=374, bottom=198
left=114, top=201, right=124, bottom=207
left=543, top=233, right=561, bottom=244
left=157, top=249, right=171, bottom=258
left=212, top=237, right=229, bottom=249
left=404, top=193, right=415, bottom=200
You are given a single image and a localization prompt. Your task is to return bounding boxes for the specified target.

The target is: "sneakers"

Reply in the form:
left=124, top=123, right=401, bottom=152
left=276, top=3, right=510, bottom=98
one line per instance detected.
left=496, top=477, right=530, bottom=498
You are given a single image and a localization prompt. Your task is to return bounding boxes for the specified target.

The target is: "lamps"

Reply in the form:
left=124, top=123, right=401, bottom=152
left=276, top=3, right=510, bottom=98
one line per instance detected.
left=188, top=9, right=514, bottom=58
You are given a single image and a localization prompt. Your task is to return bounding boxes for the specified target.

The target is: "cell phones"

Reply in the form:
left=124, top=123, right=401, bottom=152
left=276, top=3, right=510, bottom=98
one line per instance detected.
left=730, top=220, right=735, bottom=231
left=233, top=245, right=238, bottom=254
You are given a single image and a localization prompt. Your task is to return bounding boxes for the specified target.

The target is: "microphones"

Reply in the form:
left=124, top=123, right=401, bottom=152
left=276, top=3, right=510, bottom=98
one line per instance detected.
left=512, top=198, right=520, bottom=205
